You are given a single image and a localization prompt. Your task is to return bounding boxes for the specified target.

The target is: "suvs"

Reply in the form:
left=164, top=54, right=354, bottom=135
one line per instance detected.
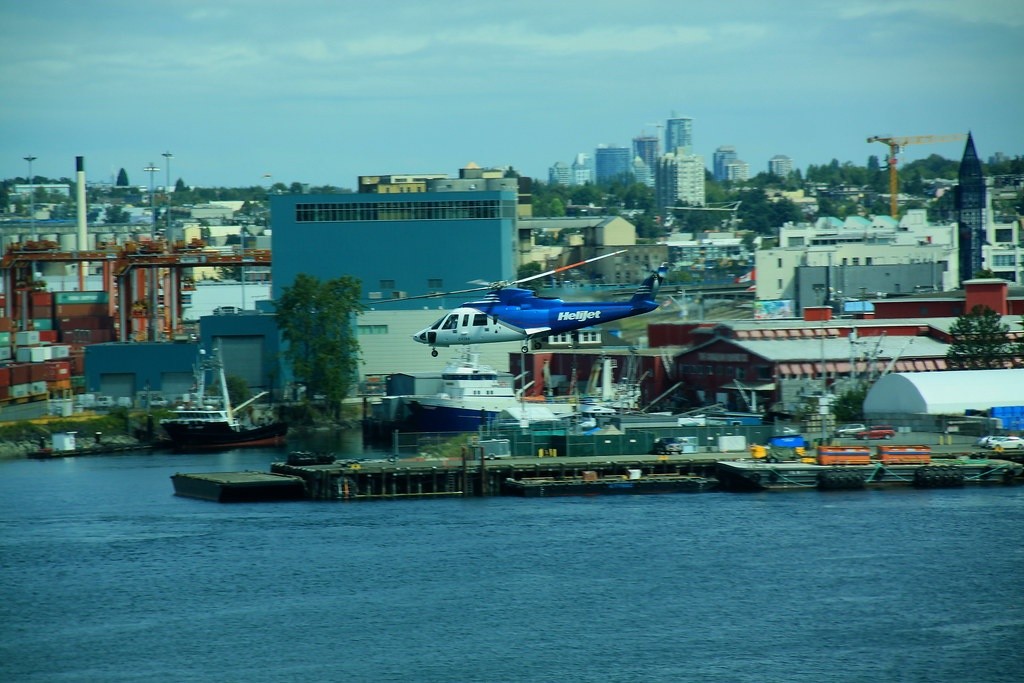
left=833, top=424, right=868, bottom=438
left=852, top=425, right=896, bottom=441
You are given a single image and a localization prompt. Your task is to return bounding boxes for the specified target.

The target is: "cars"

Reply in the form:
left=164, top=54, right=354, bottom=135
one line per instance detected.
left=987, top=436, right=1024, bottom=450
left=97, top=395, right=173, bottom=410
left=976, top=435, right=990, bottom=446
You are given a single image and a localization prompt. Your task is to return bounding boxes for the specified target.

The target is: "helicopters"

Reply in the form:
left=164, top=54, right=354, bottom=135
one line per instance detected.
left=356, top=248, right=670, bottom=356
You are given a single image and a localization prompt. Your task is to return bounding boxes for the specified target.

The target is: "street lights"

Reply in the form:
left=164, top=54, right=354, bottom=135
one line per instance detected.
left=161, top=150, right=175, bottom=255
left=144, top=162, right=161, bottom=242
left=24, top=156, right=37, bottom=240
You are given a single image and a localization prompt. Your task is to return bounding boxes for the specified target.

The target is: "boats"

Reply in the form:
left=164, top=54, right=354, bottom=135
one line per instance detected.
left=360, top=344, right=617, bottom=440
left=133, top=336, right=291, bottom=453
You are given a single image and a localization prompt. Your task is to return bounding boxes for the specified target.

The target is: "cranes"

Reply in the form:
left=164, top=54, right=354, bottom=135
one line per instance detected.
left=866, top=134, right=968, bottom=221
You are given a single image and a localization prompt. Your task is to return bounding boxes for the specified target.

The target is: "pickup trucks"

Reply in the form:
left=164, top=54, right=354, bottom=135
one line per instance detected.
left=653, top=437, right=683, bottom=455
